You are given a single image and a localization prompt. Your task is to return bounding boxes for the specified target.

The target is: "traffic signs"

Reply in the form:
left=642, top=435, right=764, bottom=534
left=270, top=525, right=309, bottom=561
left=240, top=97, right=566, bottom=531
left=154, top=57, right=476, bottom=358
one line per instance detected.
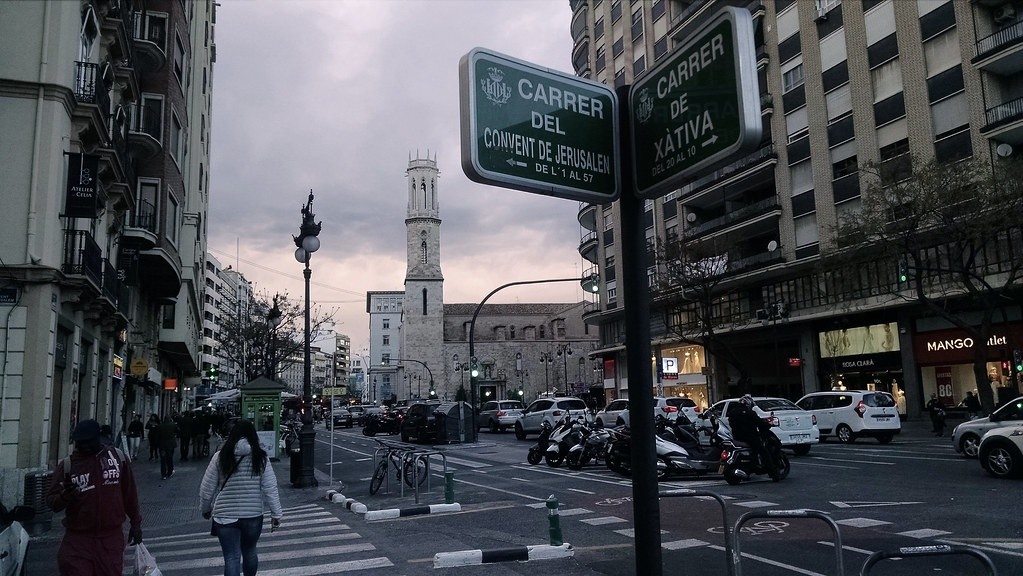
left=628, top=6, right=762, bottom=200
left=459, top=47, right=620, bottom=204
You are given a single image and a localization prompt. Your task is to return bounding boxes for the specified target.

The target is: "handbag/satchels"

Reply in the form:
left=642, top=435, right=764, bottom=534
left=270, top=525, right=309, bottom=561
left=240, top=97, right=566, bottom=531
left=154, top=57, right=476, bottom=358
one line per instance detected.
left=211, top=517, right=218, bottom=536
left=123, top=539, right=138, bottom=576
left=135, top=541, right=163, bottom=576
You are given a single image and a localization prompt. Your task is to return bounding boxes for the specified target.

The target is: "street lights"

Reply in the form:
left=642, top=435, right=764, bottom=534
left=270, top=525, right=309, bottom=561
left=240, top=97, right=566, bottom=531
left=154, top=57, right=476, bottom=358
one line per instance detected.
left=266, top=304, right=282, bottom=381
left=539, top=343, right=572, bottom=397
left=292, top=189, right=323, bottom=489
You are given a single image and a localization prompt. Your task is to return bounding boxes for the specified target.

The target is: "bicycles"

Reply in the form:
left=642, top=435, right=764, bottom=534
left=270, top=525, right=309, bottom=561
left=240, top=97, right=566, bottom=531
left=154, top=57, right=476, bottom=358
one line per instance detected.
left=369, top=439, right=428, bottom=495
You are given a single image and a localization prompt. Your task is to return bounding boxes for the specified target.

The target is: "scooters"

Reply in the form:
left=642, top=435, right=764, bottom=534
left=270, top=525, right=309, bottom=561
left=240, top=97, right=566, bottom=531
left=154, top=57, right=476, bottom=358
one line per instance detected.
left=717, top=411, right=791, bottom=484
left=527, top=405, right=730, bottom=481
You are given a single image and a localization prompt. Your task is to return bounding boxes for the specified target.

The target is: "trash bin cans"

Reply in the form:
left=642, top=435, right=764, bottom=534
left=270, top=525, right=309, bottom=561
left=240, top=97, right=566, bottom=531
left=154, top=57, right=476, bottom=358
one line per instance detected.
left=289, top=449, right=300, bottom=483
left=433, top=401, right=478, bottom=443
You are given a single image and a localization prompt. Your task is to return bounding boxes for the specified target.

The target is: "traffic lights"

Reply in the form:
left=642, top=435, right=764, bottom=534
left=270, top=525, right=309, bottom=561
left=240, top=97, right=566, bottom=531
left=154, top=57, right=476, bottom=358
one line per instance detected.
left=1013, top=350, right=1023, bottom=373
left=471, top=356, right=478, bottom=377
left=1000, top=359, right=1009, bottom=376
left=591, top=273, right=599, bottom=292
left=898, top=259, right=907, bottom=283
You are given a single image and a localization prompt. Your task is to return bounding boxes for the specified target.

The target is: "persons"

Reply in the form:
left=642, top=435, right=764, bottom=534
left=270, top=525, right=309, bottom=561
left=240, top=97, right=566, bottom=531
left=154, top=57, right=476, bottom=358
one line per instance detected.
left=927, top=392, right=947, bottom=437
left=728, top=396, right=785, bottom=481
left=957, top=392, right=982, bottom=420
left=46, top=419, right=142, bottom=576
left=198, top=419, right=282, bottom=576
left=128, top=404, right=241, bottom=478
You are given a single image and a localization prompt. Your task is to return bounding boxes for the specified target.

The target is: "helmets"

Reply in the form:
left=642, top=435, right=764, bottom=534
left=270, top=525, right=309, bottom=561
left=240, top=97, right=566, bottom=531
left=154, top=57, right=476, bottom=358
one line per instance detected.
left=738, top=395, right=756, bottom=410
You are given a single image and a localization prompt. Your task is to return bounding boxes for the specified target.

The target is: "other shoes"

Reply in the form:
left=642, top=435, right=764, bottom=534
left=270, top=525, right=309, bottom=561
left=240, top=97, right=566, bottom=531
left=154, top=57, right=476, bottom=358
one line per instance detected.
left=170, top=470, right=175, bottom=477
left=163, top=476, right=166, bottom=480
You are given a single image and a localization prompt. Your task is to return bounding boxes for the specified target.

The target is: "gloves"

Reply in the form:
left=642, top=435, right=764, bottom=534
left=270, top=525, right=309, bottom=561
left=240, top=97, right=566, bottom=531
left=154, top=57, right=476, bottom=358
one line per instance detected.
left=270, top=518, right=280, bottom=532
left=128, top=527, right=142, bottom=545
left=58, top=480, right=79, bottom=502
left=202, top=510, right=211, bottom=519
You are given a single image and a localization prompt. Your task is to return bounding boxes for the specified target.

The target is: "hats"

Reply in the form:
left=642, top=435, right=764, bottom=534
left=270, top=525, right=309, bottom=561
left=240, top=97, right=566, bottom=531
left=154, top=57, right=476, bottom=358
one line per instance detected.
left=72, top=419, right=99, bottom=441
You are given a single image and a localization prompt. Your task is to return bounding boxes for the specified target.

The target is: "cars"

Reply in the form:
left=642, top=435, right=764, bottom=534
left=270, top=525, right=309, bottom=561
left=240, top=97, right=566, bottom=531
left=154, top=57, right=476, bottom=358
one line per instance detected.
left=692, top=397, right=819, bottom=454
left=478, top=399, right=525, bottom=433
left=515, top=397, right=593, bottom=441
left=952, top=396, right=1023, bottom=477
left=794, top=390, right=902, bottom=444
left=323, top=399, right=441, bottom=443
left=594, top=397, right=701, bottom=426
